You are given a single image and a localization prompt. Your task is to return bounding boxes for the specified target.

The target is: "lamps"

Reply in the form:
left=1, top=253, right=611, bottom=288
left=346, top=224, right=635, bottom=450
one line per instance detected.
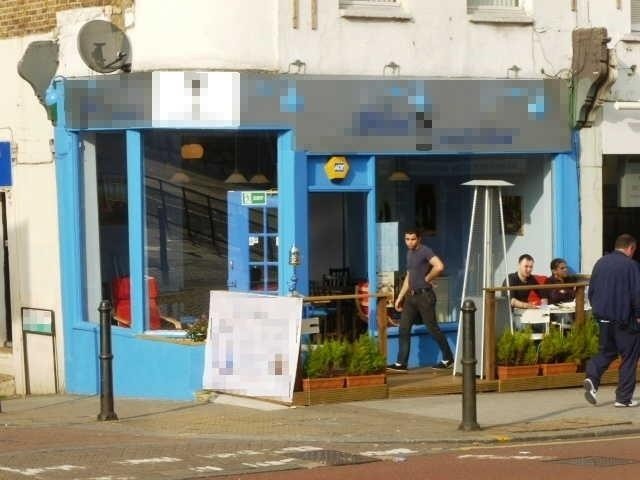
left=249, top=134, right=270, bottom=184
left=224, top=134, right=249, bottom=183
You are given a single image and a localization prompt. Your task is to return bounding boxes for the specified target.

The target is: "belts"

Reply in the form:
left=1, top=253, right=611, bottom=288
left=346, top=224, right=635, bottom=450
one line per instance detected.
left=405, top=289, right=431, bottom=297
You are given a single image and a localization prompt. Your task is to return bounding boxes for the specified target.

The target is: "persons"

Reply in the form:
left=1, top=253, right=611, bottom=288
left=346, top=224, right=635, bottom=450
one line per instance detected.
left=503, top=254, right=548, bottom=309
left=584, top=234, right=640, bottom=407
left=544, top=258, right=577, bottom=324
left=386, top=228, right=454, bottom=372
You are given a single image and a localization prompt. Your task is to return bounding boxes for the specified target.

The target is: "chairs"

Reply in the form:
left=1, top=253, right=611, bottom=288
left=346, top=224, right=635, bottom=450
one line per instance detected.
left=110, top=277, right=182, bottom=331
left=309, top=266, right=368, bottom=342
left=528, top=275, right=547, bottom=306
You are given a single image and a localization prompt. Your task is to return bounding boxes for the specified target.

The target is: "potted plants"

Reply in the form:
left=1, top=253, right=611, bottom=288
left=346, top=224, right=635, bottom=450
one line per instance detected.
left=540, top=318, right=583, bottom=375
left=346, top=332, right=388, bottom=387
left=584, top=310, right=639, bottom=369
left=496, top=321, right=539, bottom=378
left=297, top=337, right=352, bottom=392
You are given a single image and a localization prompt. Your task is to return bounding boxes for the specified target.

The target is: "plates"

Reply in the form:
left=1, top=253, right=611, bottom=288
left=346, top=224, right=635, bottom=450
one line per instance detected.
left=557, top=302, right=577, bottom=308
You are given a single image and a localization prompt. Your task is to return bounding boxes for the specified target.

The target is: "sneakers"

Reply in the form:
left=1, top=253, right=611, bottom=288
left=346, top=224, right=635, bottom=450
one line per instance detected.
left=432, top=359, right=454, bottom=371
left=614, top=399, right=640, bottom=407
left=384, top=363, right=408, bottom=373
left=583, top=378, right=598, bottom=405
